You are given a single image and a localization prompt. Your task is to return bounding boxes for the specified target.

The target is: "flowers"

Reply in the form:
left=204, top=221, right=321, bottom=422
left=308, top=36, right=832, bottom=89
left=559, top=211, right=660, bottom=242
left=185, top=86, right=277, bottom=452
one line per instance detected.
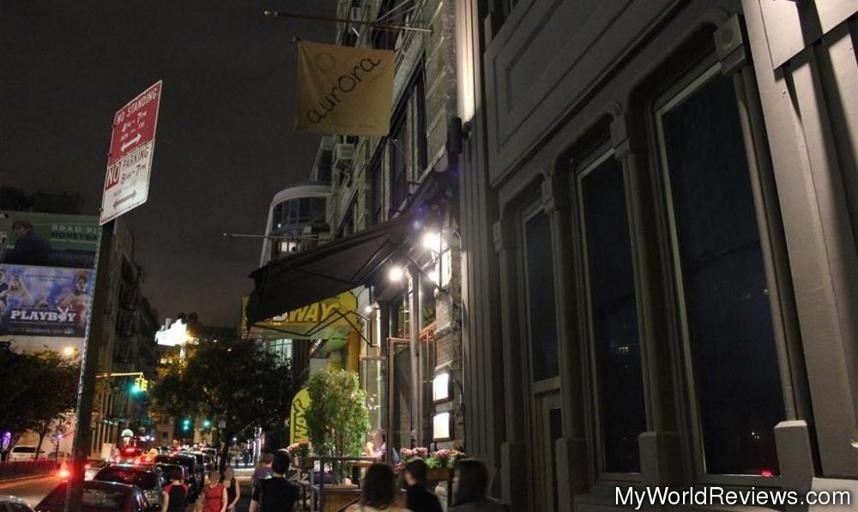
left=394, top=447, right=464, bottom=468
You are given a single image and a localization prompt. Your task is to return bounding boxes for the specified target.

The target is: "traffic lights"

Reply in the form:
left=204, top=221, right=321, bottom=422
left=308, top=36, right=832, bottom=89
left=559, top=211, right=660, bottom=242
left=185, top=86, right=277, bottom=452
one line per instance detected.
left=141, top=378, right=149, bottom=392
left=203, top=417, right=211, bottom=432
left=134, top=378, right=140, bottom=393
left=183, top=417, right=191, bottom=432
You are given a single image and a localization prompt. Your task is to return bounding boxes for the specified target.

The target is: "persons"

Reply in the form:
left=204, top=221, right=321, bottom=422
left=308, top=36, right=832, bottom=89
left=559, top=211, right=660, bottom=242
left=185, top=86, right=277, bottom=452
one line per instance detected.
left=161, top=445, right=298, bottom=511
left=343, top=463, right=413, bottom=512
left=0, top=268, right=92, bottom=330
left=402, top=459, right=445, bottom=510
left=447, top=455, right=514, bottom=512
left=8, top=219, right=59, bottom=254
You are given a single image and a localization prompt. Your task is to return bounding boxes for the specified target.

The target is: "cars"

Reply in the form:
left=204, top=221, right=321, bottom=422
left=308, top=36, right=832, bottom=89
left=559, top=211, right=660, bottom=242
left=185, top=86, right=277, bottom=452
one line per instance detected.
left=36, top=441, right=218, bottom=511
left=0, top=491, right=37, bottom=512
left=10, top=444, right=44, bottom=461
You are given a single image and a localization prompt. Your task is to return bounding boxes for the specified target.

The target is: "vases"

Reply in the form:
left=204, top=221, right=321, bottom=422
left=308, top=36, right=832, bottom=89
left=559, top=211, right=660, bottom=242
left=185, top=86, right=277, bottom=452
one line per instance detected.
left=426, top=466, right=450, bottom=482
